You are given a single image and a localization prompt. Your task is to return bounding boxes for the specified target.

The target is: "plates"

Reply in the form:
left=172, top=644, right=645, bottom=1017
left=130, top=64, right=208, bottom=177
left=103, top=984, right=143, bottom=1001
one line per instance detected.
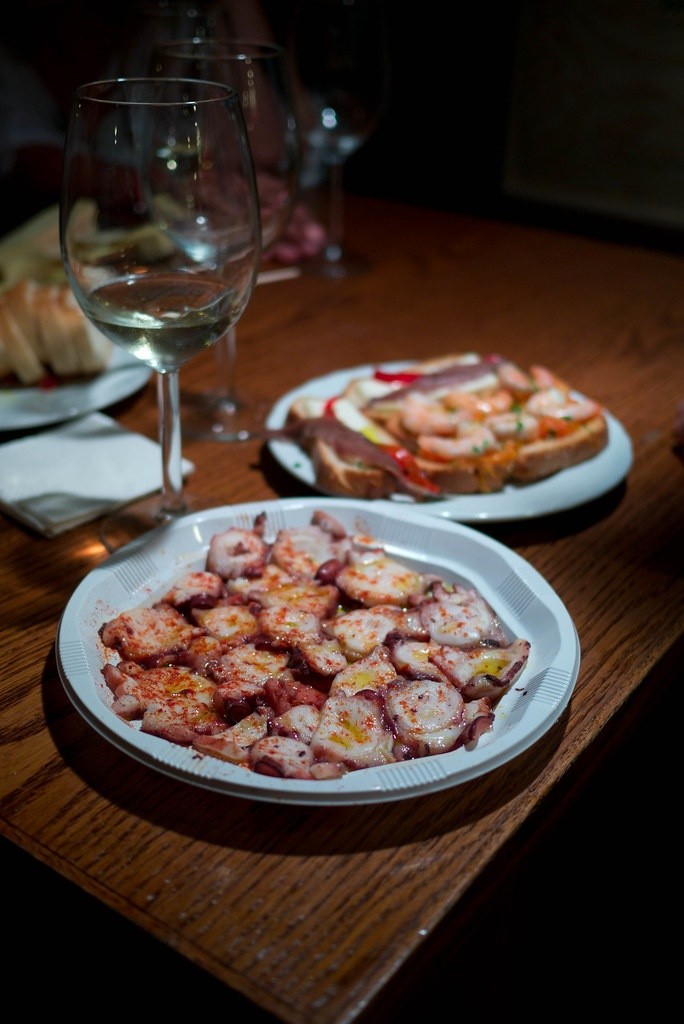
left=57, top=499, right=581, bottom=802
left=266, top=363, right=635, bottom=519
left=0, top=288, right=159, bottom=430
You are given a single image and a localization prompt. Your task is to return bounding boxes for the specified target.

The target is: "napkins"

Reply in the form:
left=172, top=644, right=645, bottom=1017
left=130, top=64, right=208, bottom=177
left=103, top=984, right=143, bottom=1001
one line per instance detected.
left=0, top=410, right=194, bottom=539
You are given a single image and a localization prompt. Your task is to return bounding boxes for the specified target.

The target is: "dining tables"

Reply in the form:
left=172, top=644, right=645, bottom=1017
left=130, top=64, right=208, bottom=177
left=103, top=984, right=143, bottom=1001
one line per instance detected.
left=0, top=189, right=684, bottom=1024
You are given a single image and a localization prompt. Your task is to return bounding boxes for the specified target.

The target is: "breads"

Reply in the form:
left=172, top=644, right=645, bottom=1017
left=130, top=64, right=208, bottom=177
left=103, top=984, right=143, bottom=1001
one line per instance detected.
left=0, top=281, right=114, bottom=381
left=290, top=369, right=606, bottom=493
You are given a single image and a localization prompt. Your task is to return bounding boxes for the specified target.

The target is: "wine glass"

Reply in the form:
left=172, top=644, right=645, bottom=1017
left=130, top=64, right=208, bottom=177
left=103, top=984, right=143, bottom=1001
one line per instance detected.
left=296, top=88, right=372, bottom=275
left=59, top=76, right=259, bottom=550
left=153, top=37, right=294, bottom=441
